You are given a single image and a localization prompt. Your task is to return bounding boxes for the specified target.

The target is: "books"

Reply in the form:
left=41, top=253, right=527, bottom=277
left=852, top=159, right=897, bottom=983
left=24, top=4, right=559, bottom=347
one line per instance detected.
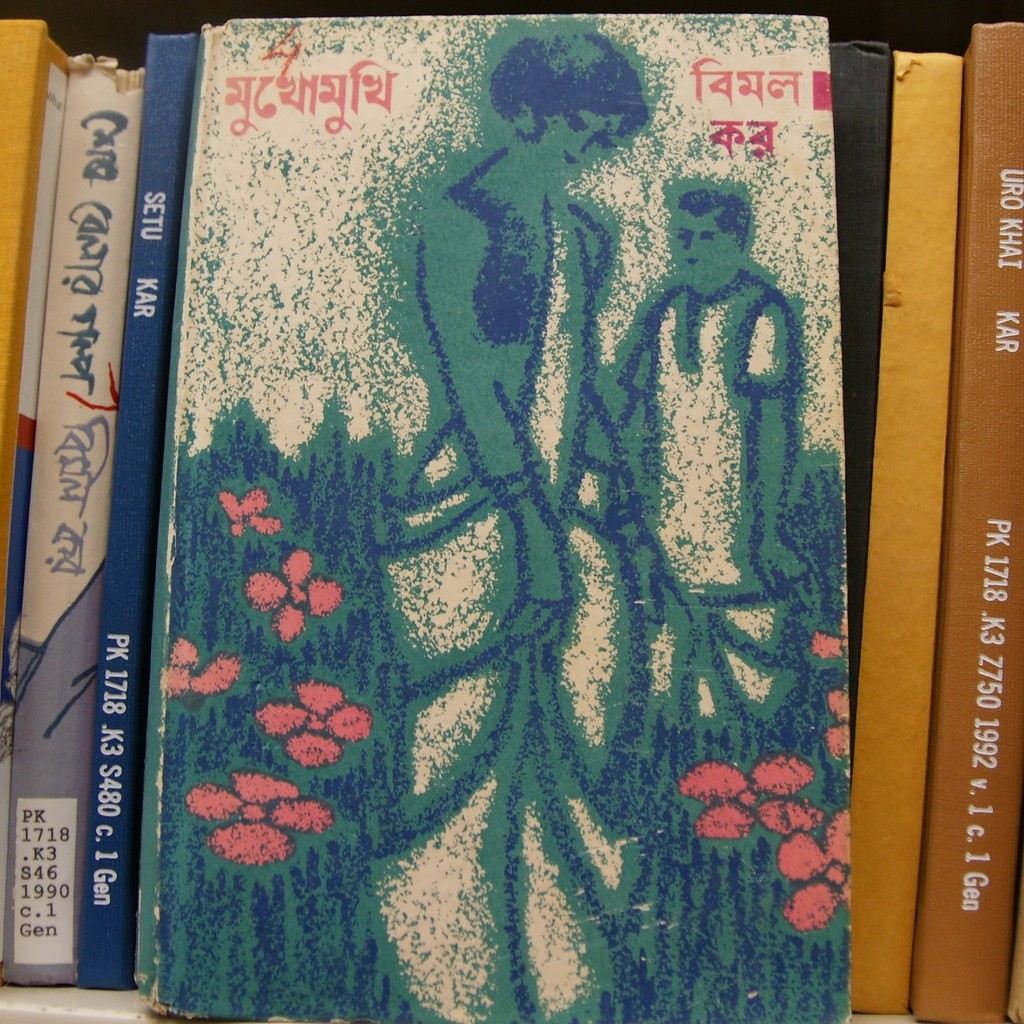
left=2, top=13, right=1024, bottom=1024
